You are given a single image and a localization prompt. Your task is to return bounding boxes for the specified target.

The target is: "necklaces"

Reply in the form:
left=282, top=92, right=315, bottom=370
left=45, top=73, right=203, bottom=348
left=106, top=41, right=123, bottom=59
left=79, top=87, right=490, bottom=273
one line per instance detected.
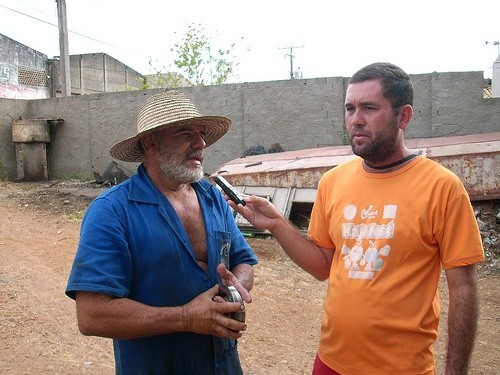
left=362, top=153, right=418, bottom=170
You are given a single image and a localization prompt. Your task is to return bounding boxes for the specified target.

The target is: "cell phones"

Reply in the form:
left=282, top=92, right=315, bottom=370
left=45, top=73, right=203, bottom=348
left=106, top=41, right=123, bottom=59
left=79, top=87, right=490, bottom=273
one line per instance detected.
left=213, top=176, right=246, bottom=207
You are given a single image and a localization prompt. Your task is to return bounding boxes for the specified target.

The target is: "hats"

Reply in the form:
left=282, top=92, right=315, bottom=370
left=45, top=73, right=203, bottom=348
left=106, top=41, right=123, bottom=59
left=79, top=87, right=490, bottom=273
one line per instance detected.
left=108, top=90, right=232, bottom=162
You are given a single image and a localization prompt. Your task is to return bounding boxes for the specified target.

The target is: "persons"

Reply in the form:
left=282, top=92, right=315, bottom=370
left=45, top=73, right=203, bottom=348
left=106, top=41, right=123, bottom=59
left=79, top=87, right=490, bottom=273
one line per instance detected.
left=64, top=90, right=259, bottom=375
left=220, top=62, right=485, bottom=375
